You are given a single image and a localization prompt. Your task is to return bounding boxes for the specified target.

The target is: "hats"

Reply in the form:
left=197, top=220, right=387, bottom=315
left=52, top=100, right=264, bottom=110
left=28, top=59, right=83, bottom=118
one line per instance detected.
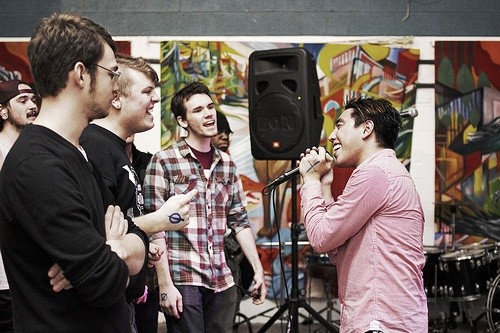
left=0, top=79, right=38, bottom=106
left=216, top=108, right=233, bottom=132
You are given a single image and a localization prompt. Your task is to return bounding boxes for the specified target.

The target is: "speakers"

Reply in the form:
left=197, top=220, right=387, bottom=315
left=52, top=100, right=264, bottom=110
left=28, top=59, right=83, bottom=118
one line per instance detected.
left=248, top=47, right=324, bottom=160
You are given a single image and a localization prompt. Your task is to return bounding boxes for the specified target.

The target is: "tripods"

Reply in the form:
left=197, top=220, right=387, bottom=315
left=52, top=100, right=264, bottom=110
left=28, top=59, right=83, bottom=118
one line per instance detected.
left=257, top=160, right=339, bottom=333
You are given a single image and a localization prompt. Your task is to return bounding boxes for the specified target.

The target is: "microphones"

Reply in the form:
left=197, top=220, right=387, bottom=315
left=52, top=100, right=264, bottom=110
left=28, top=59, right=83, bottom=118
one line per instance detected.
left=266, top=151, right=330, bottom=189
left=399, top=108, right=418, bottom=118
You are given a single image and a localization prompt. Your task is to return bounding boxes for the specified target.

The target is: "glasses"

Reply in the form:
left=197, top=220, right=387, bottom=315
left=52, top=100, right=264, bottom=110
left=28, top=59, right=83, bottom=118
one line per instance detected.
left=92, top=61, right=123, bottom=82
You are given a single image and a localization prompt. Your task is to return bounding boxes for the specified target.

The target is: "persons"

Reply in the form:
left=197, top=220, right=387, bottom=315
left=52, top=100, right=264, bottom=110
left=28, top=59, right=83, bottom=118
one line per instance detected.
left=0, top=12, right=267, bottom=333
left=296, top=97, right=428, bottom=333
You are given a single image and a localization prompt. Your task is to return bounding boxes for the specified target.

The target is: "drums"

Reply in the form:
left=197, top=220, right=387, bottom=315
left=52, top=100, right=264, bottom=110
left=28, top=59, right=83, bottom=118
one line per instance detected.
left=440, top=250, right=487, bottom=301
left=423, top=247, right=441, bottom=302
left=486, top=274, right=500, bottom=333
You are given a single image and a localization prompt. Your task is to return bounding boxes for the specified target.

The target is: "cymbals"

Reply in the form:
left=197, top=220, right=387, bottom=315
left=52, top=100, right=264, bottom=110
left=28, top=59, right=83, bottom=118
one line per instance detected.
left=257, top=241, right=310, bottom=246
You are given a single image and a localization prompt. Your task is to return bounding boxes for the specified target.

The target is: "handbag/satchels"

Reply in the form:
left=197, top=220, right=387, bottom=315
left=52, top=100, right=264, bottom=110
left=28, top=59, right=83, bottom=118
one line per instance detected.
left=223, top=232, right=255, bottom=313
